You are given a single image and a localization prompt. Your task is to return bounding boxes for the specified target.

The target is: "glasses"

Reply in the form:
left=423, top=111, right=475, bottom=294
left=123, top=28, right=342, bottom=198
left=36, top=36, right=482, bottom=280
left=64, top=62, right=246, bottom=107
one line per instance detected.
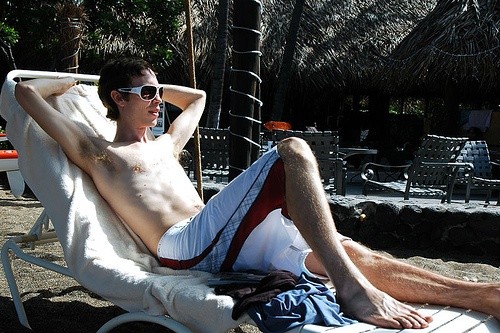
left=117, top=85, right=164, bottom=102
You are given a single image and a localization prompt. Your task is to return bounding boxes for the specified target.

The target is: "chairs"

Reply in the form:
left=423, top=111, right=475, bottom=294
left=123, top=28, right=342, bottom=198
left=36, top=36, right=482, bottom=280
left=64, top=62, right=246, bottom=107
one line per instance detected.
left=186, top=124, right=231, bottom=185
left=362, top=134, right=468, bottom=204
left=445, top=140, right=500, bottom=208
left=0, top=70, right=500, bottom=333
left=269, top=126, right=378, bottom=195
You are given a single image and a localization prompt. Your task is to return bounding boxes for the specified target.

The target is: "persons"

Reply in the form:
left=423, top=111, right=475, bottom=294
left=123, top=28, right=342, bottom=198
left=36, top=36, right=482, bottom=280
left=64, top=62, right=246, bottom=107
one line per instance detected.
left=467, top=127, right=482, bottom=140
left=15, top=58, right=500, bottom=329
